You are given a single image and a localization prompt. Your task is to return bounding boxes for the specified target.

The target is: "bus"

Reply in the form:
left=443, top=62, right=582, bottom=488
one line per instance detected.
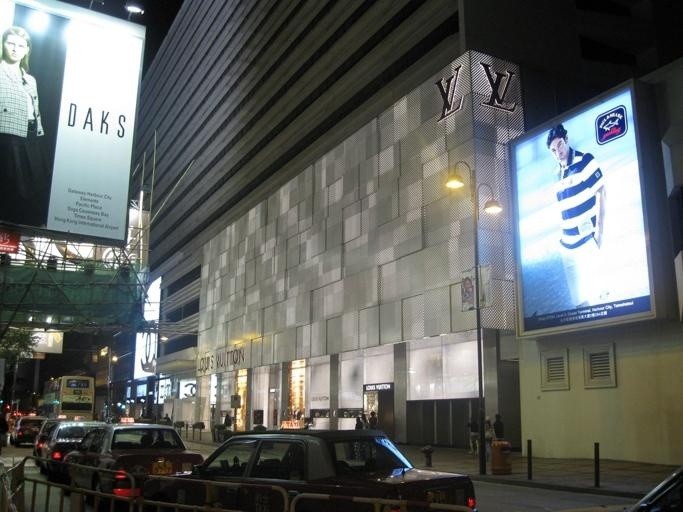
left=38, top=376, right=97, bottom=421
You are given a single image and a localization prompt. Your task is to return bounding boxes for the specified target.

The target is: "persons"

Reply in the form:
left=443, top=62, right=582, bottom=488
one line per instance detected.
left=355, top=412, right=378, bottom=429
left=467, top=413, right=504, bottom=456
left=164, top=413, right=171, bottom=423
left=0, top=27, right=45, bottom=226
left=545, top=123, right=608, bottom=307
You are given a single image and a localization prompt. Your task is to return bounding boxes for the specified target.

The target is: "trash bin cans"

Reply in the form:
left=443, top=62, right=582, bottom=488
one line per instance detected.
left=491, top=440, right=513, bottom=475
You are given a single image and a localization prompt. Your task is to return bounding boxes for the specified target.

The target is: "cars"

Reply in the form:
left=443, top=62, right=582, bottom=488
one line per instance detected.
left=10, top=416, right=44, bottom=446
left=64, top=422, right=204, bottom=510
left=34, top=419, right=88, bottom=477
left=143, top=430, right=477, bottom=507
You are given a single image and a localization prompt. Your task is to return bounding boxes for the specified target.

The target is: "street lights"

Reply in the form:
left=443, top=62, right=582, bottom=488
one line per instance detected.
left=445, top=161, right=504, bottom=476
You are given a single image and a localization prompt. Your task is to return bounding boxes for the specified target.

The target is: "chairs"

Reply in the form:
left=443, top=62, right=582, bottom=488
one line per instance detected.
left=137, top=434, right=154, bottom=447
left=260, top=446, right=385, bottom=477
